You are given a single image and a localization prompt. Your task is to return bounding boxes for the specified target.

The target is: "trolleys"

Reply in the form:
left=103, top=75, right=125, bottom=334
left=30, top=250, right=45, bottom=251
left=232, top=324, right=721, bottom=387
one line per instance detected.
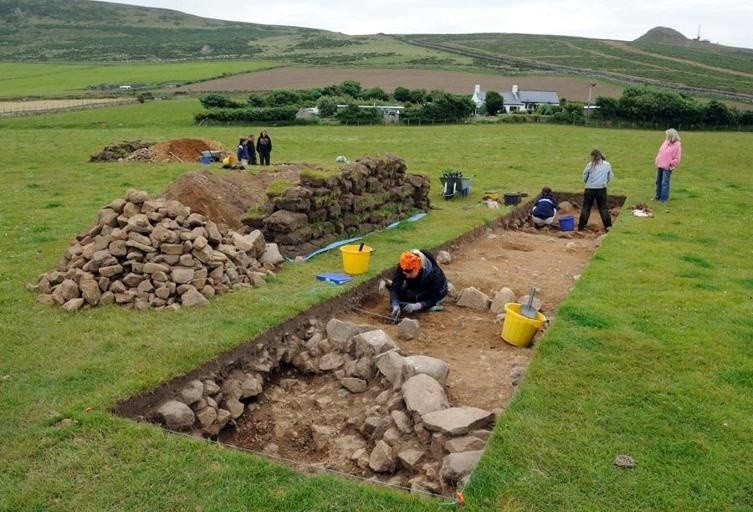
left=439, top=170, right=477, bottom=200
left=195, top=148, right=221, bottom=162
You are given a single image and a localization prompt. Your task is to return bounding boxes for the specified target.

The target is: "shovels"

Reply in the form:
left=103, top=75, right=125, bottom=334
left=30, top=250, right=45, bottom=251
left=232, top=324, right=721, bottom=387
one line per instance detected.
left=519, top=288, right=537, bottom=319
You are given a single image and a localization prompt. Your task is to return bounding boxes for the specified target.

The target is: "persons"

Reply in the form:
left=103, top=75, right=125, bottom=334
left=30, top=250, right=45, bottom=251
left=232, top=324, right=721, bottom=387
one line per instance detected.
left=654, top=128, right=683, bottom=203
left=247, top=134, right=257, bottom=165
left=532, top=186, right=562, bottom=227
left=578, top=149, right=614, bottom=231
left=238, top=138, right=250, bottom=165
left=389, top=246, right=448, bottom=324
left=256, top=130, right=272, bottom=165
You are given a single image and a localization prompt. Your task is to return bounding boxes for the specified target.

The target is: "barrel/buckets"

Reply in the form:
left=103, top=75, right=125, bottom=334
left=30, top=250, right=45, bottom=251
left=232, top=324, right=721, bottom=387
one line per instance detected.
left=340, top=245, right=372, bottom=275
left=222, top=156, right=236, bottom=167
left=501, top=303, right=547, bottom=348
left=558, top=215, right=575, bottom=231
left=504, top=192, right=520, bottom=206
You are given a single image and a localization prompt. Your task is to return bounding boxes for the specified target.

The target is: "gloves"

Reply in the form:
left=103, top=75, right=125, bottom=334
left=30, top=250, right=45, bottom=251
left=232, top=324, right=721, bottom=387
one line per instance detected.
left=391, top=305, right=400, bottom=319
left=403, top=302, right=422, bottom=313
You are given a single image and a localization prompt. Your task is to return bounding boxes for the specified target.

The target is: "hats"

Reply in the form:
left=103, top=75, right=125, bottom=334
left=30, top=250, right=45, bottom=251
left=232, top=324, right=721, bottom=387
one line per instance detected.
left=399, top=251, right=419, bottom=270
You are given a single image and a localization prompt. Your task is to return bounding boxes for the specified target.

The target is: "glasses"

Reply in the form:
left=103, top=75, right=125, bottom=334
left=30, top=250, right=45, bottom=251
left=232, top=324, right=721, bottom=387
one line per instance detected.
left=399, top=266, right=415, bottom=273
